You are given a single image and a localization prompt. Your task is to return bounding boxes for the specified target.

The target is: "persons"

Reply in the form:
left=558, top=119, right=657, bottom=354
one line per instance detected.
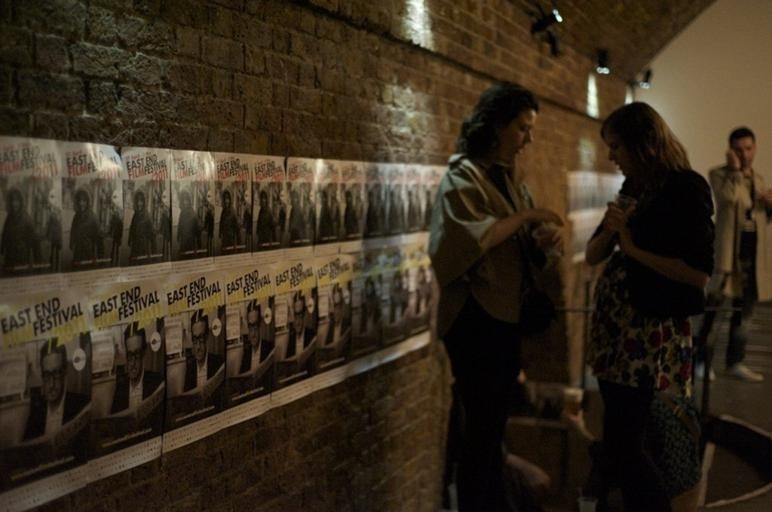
left=429, top=82, right=564, bottom=510
left=1, top=185, right=431, bottom=441
left=586, top=102, right=715, bottom=510
left=709, top=126, right=771, bottom=382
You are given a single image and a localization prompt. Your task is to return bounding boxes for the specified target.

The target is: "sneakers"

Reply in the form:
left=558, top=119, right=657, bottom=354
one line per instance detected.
left=694, top=362, right=715, bottom=381
left=725, top=362, right=764, bottom=384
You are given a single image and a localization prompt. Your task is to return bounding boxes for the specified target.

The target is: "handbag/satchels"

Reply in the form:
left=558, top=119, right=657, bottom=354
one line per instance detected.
left=517, top=289, right=559, bottom=336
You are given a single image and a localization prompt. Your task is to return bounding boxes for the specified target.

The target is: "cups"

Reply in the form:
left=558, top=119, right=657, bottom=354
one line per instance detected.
left=563, top=387, right=585, bottom=414
left=603, top=193, right=638, bottom=236
left=531, top=221, right=568, bottom=266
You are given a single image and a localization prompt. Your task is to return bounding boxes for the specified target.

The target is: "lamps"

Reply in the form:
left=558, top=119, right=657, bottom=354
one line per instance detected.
left=525, top=0, right=653, bottom=94
